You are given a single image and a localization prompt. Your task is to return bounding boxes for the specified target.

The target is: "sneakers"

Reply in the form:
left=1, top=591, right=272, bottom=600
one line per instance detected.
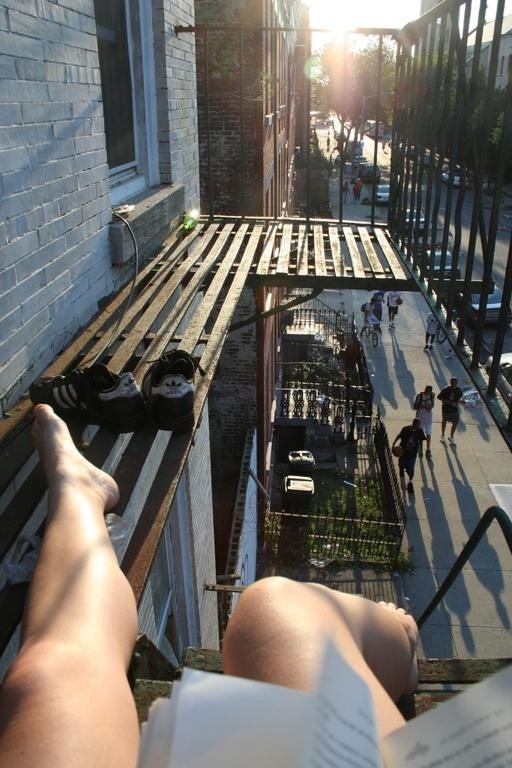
left=447, top=434, right=457, bottom=446
left=423, top=343, right=435, bottom=351
left=140, top=347, right=208, bottom=434
left=388, top=322, right=396, bottom=330
left=440, top=433, right=446, bottom=442
left=26, top=361, right=148, bottom=436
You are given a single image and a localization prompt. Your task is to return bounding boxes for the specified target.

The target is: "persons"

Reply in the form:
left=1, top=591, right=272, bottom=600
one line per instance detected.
left=387, top=290, right=400, bottom=330
left=392, top=419, right=426, bottom=493
left=414, top=385, right=435, bottom=460
left=359, top=297, right=381, bottom=337
left=423, top=310, right=439, bottom=351
left=436, top=377, right=463, bottom=445
left=0, top=403, right=420, bottom=768
left=342, top=178, right=363, bottom=204
left=373, top=289, right=386, bottom=321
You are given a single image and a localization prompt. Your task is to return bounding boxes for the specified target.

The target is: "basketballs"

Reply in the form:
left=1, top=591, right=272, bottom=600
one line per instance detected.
left=393, top=446, right=404, bottom=457
left=396, top=299, right=402, bottom=304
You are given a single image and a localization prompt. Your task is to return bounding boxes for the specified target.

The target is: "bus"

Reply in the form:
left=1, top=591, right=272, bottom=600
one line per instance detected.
left=365, top=119, right=386, bottom=138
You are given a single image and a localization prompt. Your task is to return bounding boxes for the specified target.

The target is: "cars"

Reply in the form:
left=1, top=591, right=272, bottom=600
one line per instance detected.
left=456, top=279, right=512, bottom=328
left=346, top=135, right=472, bottom=207
left=485, top=351, right=512, bottom=410
left=399, top=208, right=462, bottom=282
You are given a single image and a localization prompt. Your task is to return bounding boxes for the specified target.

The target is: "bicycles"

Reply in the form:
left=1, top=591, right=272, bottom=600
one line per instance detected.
left=363, top=320, right=380, bottom=347
left=434, top=320, right=447, bottom=343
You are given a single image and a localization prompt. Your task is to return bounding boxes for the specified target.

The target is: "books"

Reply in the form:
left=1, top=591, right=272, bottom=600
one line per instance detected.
left=136, top=639, right=511, bottom=768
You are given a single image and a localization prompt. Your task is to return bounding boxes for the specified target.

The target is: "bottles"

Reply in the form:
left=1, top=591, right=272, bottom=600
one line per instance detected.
left=183, top=210, right=199, bottom=230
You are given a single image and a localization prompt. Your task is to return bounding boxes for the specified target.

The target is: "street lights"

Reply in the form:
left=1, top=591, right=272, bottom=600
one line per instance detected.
left=358, top=91, right=396, bottom=142
